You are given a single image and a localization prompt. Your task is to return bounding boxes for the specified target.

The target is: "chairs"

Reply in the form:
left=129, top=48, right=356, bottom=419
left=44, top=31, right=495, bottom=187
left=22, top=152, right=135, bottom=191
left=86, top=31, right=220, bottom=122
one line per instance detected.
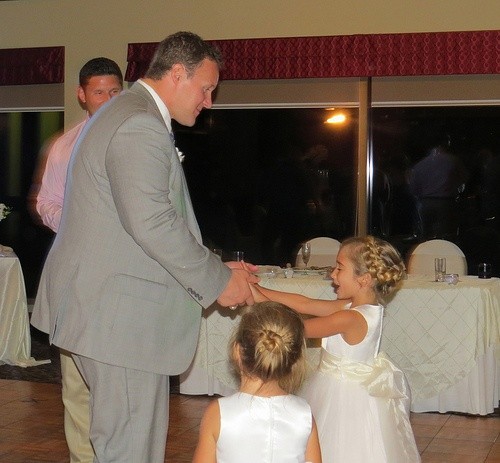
left=407, top=239, right=468, bottom=277
left=294, top=235, right=342, bottom=268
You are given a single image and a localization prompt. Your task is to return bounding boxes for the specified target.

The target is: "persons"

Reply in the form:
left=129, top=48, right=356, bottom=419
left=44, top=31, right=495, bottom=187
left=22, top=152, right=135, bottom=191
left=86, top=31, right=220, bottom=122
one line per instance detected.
left=181, top=302, right=323, bottom=463
left=242, top=235, right=421, bottom=463
left=37, top=57, right=124, bottom=463
left=29, top=31, right=260, bottom=463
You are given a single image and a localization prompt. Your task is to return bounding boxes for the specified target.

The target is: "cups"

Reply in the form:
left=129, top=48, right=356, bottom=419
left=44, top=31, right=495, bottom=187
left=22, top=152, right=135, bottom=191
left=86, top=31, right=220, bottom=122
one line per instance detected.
left=434, top=257, right=447, bottom=282
left=212, top=248, right=223, bottom=262
left=233, top=250, right=244, bottom=262
left=444, top=274, right=460, bottom=285
left=479, top=264, right=492, bottom=279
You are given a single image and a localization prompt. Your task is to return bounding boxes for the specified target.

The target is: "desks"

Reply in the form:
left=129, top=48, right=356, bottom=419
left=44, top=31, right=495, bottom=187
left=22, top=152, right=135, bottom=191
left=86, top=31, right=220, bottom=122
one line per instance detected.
left=178, top=266, right=500, bottom=417
left=0, top=246, right=52, bottom=369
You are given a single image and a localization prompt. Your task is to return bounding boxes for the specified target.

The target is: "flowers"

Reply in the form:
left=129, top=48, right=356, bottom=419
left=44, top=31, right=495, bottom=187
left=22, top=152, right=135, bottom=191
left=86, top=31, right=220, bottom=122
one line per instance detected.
left=0, top=203, right=14, bottom=222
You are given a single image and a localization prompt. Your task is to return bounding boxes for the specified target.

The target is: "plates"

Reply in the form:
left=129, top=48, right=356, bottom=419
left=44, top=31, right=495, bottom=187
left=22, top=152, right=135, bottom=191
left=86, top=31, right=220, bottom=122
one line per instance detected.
left=252, top=265, right=338, bottom=277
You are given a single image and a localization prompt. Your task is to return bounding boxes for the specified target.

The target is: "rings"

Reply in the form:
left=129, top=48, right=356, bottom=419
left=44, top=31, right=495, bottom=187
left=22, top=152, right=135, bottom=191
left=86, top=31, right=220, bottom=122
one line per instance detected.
left=229, top=304, right=238, bottom=311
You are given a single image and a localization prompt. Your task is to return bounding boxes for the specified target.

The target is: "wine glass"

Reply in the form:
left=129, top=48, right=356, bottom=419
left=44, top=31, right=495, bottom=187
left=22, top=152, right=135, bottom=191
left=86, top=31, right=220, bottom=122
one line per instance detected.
left=301, top=243, right=311, bottom=268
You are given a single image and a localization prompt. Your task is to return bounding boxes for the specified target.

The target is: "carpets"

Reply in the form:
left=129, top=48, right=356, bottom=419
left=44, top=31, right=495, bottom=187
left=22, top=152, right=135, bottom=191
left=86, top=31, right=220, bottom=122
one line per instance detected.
left=0, top=336, right=181, bottom=394
